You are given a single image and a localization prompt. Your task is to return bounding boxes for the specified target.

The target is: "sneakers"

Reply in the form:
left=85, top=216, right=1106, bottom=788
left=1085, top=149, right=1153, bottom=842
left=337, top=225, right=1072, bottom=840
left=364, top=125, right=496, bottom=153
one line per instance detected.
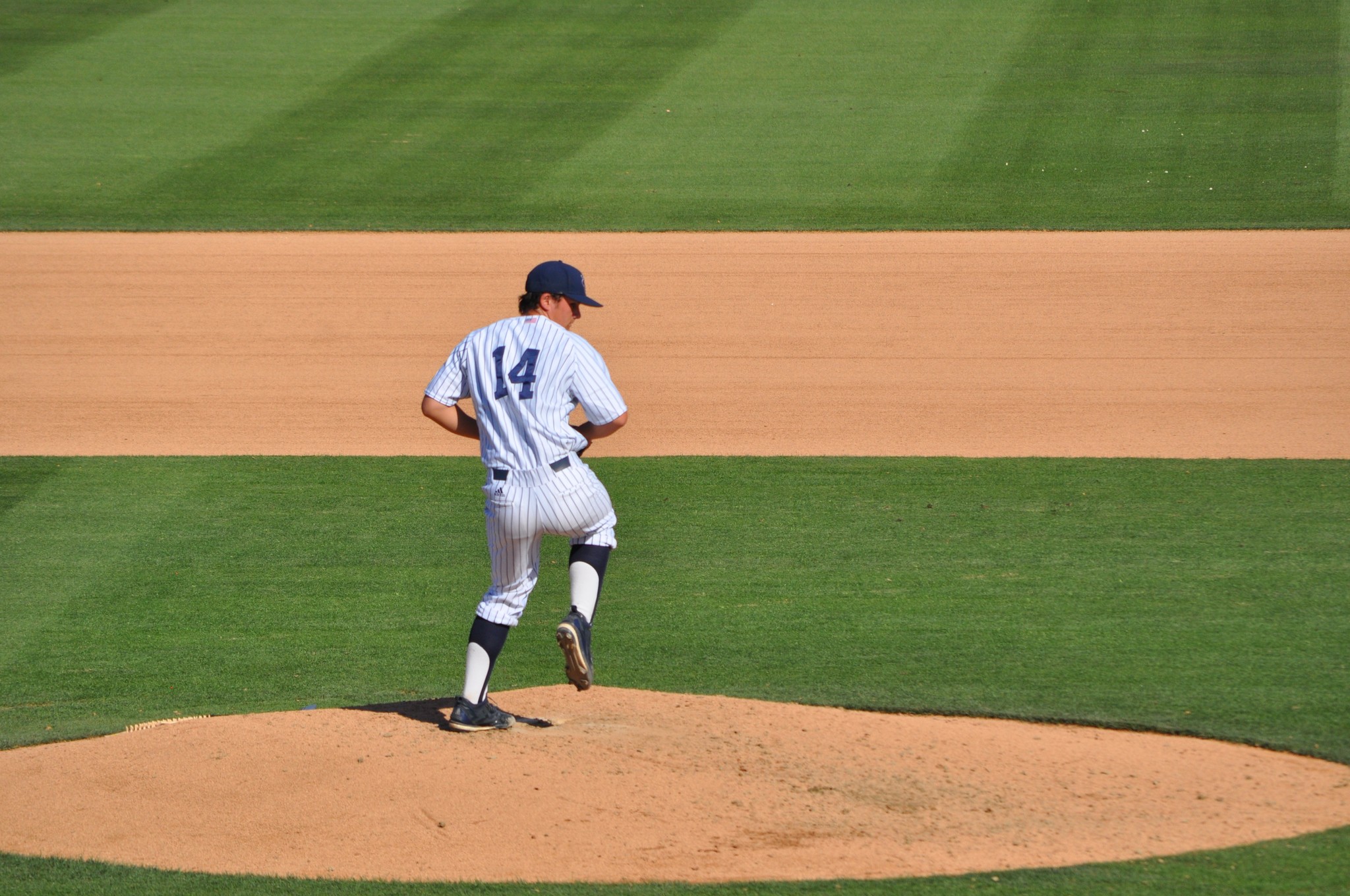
left=556, top=605, right=593, bottom=692
left=449, top=691, right=517, bottom=731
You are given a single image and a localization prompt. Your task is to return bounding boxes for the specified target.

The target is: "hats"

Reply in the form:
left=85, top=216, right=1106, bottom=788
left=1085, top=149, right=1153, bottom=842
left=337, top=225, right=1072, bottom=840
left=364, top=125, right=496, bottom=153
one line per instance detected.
left=526, top=260, right=603, bottom=306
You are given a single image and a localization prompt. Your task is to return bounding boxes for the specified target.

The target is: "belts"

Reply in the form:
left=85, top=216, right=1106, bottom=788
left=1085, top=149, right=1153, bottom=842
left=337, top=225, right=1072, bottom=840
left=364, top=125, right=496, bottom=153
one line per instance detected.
left=493, top=456, right=570, bottom=481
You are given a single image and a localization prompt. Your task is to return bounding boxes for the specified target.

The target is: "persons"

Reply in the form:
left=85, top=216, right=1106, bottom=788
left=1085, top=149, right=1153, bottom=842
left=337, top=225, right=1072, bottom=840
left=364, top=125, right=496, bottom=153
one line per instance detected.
left=421, top=260, right=628, bottom=732
